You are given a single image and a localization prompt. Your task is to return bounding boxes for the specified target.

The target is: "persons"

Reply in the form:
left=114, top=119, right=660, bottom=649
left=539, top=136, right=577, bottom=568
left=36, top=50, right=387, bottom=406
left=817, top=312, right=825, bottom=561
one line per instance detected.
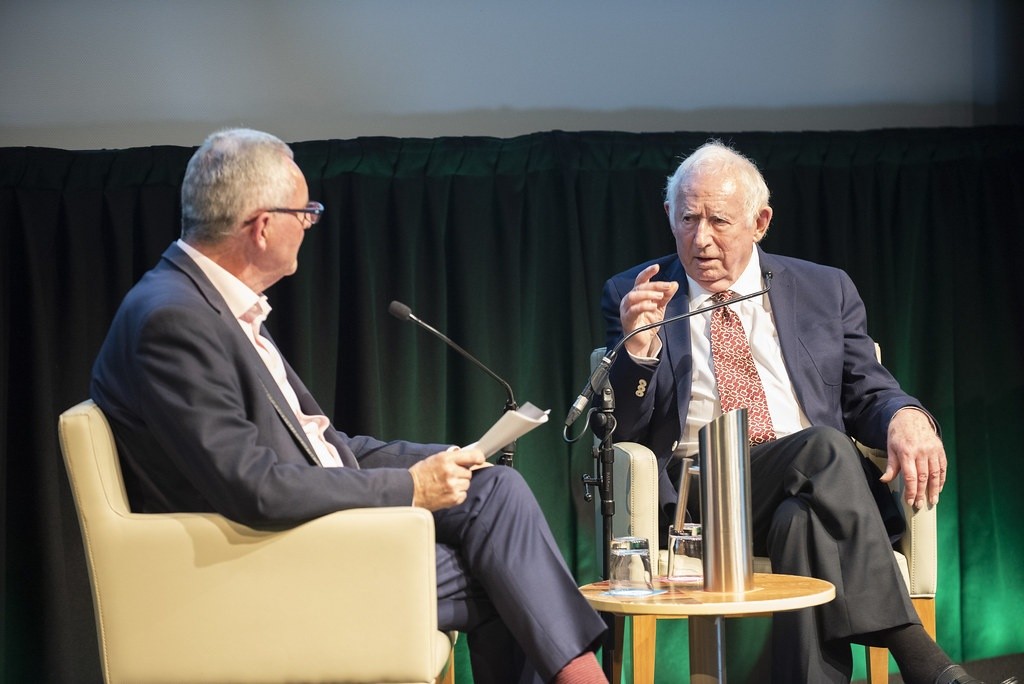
left=594, top=138, right=1021, bottom=684
left=87, top=128, right=610, bottom=683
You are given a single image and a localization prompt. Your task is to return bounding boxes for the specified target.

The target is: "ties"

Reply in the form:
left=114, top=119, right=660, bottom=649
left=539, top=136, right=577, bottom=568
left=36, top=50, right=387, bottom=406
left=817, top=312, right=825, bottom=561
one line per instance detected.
left=710, top=291, right=776, bottom=447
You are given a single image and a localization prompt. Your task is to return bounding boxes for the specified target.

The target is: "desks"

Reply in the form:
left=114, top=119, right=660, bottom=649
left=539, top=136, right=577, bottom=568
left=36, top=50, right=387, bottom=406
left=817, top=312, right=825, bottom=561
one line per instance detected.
left=579, top=573, right=836, bottom=684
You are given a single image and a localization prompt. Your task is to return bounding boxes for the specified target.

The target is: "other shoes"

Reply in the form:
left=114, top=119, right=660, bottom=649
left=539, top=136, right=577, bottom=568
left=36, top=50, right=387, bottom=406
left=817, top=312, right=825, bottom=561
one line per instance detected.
left=937, top=663, right=1022, bottom=684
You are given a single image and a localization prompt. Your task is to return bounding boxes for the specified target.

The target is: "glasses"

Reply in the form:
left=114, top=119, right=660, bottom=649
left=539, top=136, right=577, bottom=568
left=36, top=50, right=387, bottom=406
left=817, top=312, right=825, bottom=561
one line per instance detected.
left=244, top=201, right=324, bottom=226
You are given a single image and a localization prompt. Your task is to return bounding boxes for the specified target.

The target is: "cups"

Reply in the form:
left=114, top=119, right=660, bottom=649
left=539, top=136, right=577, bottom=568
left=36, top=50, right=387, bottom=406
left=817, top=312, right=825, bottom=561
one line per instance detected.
left=668, top=523, right=703, bottom=581
left=609, top=537, right=654, bottom=596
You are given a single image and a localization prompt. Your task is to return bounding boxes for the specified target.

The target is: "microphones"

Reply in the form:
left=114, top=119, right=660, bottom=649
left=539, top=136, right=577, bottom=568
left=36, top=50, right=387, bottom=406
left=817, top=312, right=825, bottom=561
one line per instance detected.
left=387, top=301, right=518, bottom=468
left=562, top=266, right=773, bottom=426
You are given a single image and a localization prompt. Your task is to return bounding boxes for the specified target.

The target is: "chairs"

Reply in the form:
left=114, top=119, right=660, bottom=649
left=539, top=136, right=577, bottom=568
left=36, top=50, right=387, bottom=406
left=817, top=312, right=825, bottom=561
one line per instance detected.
left=589, top=343, right=937, bottom=684
left=57, top=392, right=461, bottom=684
